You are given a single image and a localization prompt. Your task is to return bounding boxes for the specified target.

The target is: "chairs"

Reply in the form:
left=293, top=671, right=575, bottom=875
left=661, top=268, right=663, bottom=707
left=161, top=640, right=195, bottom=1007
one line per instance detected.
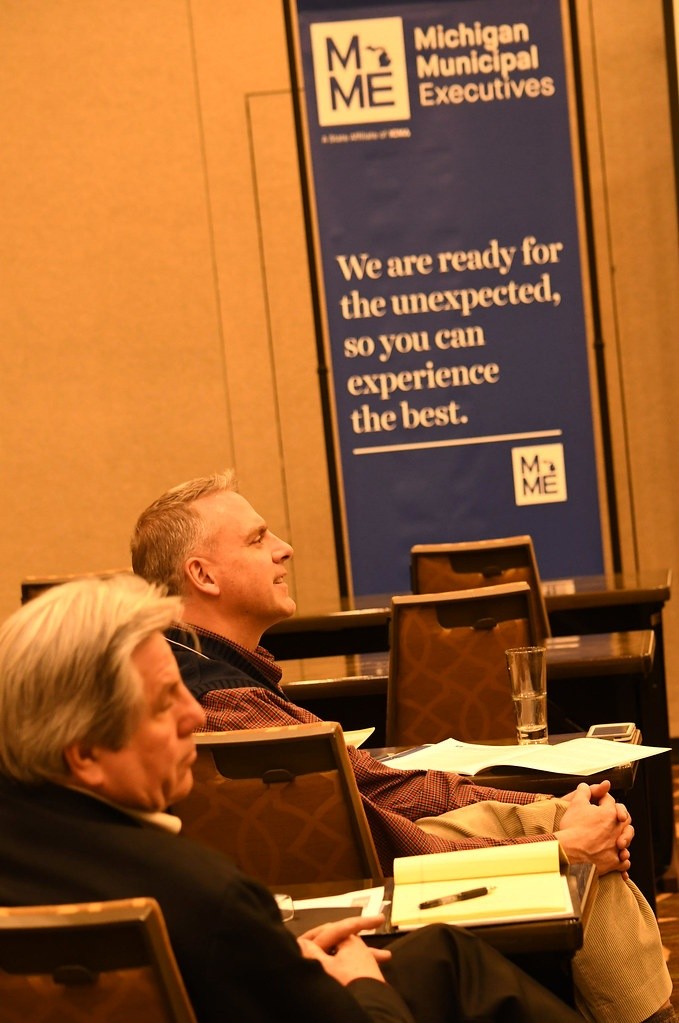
left=0, top=534, right=552, bottom=1023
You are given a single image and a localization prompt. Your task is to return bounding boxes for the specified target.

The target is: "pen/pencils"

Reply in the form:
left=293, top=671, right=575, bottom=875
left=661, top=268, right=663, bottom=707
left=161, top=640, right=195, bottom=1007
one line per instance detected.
left=419, top=884, right=498, bottom=910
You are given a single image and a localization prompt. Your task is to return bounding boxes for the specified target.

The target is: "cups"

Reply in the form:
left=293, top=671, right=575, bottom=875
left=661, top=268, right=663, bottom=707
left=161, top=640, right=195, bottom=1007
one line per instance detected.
left=504, top=646, right=548, bottom=744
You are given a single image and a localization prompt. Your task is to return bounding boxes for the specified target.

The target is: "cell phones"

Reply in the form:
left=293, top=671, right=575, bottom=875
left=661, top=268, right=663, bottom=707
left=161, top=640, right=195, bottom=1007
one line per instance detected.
left=587, top=722, right=636, bottom=742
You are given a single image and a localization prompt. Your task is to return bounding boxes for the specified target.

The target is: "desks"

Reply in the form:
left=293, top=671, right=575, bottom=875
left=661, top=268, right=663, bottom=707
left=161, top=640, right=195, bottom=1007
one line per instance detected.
left=259, top=568, right=673, bottom=866
left=359, top=732, right=642, bottom=808
left=262, top=865, right=599, bottom=1009
left=274, top=629, right=657, bottom=919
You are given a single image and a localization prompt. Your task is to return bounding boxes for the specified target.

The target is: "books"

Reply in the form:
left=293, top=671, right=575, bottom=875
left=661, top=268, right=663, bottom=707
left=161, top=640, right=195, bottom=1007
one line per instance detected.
left=278, top=907, right=364, bottom=951
left=369, top=737, right=672, bottom=778
left=390, top=839, right=581, bottom=930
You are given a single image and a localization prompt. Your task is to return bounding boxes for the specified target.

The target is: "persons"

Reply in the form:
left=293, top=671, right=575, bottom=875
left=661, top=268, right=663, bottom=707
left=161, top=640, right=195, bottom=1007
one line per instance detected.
left=0, top=573, right=585, bottom=1023
left=132, top=473, right=679, bottom=1023
